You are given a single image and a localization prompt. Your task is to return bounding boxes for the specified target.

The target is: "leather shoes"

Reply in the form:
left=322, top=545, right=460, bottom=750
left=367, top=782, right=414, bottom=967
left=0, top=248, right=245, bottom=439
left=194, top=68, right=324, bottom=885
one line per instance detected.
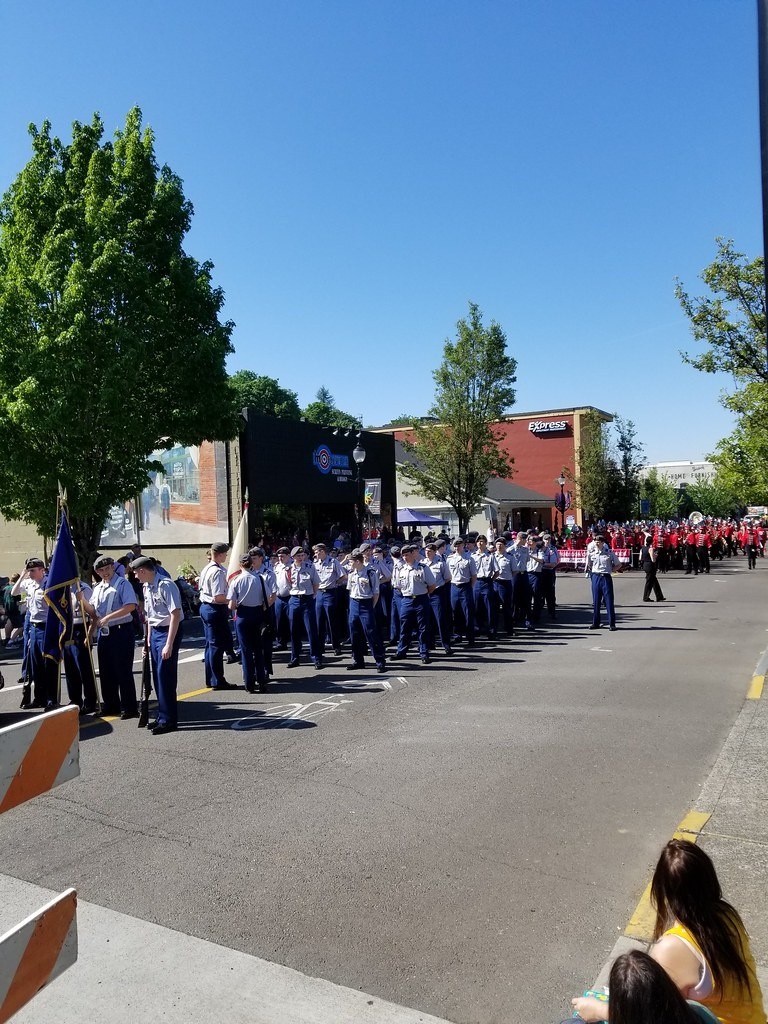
left=20, top=698, right=60, bottom=712
left=121, top=711, right=139, bottom=720
left=589, top=624, right=601, bottom=629
left=609, top=626, right=617, bottom=631
left=202, top=607, right=557, bottom=694
left=147, top=720, right=178, bottom=734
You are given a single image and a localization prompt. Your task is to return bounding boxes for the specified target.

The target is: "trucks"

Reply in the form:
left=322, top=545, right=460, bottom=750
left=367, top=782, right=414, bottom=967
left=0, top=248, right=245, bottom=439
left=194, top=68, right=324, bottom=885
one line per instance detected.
left=746, top=505, right=768, bottom=519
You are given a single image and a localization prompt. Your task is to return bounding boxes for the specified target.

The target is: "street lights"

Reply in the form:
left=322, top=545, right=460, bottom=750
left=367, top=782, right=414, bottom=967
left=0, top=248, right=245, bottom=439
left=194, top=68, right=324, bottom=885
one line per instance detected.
left=353, top=438, right=366, bottom=548
left=557, top=472, right=566, bottom=527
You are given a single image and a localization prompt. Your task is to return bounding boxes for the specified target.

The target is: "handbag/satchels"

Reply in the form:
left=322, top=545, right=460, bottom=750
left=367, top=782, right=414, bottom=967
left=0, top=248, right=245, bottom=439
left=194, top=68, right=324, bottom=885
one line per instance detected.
left=258, top=622, right=276, bottom=641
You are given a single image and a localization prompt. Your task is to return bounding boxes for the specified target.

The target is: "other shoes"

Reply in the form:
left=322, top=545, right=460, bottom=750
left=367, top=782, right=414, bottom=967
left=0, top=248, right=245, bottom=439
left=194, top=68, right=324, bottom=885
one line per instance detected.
left=643, top=598, right=654, bottom=602
left=67, top=701, right=97, bottom=716
left=656, top=552, right=765, bottom=575
left=656, top=598, right=666, bottom=602
left=17, top=676, right=28, bottom=683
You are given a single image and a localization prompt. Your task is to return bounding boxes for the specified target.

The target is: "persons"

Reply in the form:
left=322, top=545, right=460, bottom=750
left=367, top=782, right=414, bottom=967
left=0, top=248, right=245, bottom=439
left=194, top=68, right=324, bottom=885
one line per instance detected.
left=11, top=557, right=59, bottom=712
left=568, top=839, right=768, bottom=1023
left=250, top=519, right=565, bottom=672
left=638, top=536, right=666, bottom=602
left=63, top=578, right=97, bottom=715
left=607, top=948, right=721, bottom=1024
left=128, top=557, right=185, bottom=734
left=76, top=555, right=140, bottom=721
left=225, top=552, right=275, bottom=694
left=0, top=572, right=32, bottom=682
left=197, top=541, right=238, bottom=691
left=248, top=546, right=280, bottom=678
left=566, top=517, right=768, bottom=575
left=114, top=544, right=173, bottom=638
left=583, top=531, right=622, bottom=632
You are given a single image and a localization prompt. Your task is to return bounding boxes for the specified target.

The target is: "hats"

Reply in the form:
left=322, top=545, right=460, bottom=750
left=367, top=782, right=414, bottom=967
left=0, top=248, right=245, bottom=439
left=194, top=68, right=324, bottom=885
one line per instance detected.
left=94, top=555, right=114, bottom=570
left=594, top=535, right=605, bottom=542
left=207, top=529, right=551, bottom=560
left=570, top=515, right=763, bottom=534
left=129, top=557, right=154, bottom=570
left=25, top=557, right=44, bottom=569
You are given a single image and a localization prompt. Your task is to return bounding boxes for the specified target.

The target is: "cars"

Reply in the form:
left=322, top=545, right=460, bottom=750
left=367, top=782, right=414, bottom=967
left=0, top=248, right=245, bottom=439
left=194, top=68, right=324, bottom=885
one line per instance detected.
left=740, top=514, right=764, bottom=528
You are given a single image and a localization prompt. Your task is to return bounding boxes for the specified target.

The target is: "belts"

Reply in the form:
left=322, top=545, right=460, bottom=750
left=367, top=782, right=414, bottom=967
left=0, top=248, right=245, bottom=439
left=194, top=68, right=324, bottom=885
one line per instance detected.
left=319, top=589, right=333, bottom=593
left=407, top=595, right=419, bottom=599
left=295, top=595, right=311, bottom=599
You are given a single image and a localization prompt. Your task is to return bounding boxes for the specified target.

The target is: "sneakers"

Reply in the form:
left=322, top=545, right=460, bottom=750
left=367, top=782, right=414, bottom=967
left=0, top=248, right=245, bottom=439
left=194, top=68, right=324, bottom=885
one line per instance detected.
left=5, top=637, right=24, bottom=649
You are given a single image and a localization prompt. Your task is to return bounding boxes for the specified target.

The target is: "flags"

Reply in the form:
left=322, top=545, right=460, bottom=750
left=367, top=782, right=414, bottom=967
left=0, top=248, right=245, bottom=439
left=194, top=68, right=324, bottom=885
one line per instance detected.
left=42, top=508, right=81, bottom=664
left=226, top=499, right=249, bottom=581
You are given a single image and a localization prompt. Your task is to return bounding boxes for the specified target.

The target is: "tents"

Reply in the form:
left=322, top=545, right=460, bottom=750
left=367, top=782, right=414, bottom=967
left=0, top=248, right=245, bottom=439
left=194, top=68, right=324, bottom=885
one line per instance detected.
left=396, top=507, right=448, bottom=542
